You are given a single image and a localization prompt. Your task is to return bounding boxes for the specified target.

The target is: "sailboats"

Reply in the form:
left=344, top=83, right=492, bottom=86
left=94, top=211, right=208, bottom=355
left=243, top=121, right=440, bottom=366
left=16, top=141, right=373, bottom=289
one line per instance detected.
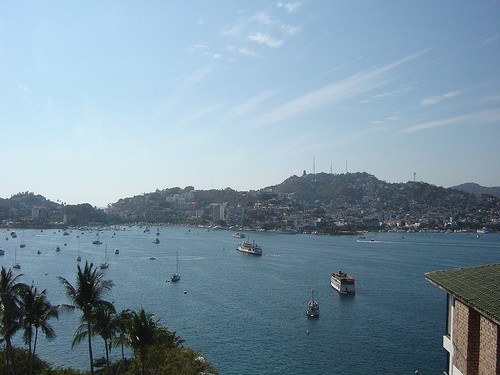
left=171, top=250, right=181, bottom=280
left=76, top=239, right=82, bottom=261
left=100, top=243, right=110, bottom=269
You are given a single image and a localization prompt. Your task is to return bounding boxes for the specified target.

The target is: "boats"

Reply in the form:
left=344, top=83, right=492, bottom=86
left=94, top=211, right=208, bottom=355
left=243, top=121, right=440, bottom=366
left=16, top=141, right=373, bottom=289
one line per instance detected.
left=330, top=270, right=355, bottom=294
left=233, top=232, right=245, bottom=238
left=236, top=236, right=262, bottom=255
left=306, top=289, right=321, bottom=317
